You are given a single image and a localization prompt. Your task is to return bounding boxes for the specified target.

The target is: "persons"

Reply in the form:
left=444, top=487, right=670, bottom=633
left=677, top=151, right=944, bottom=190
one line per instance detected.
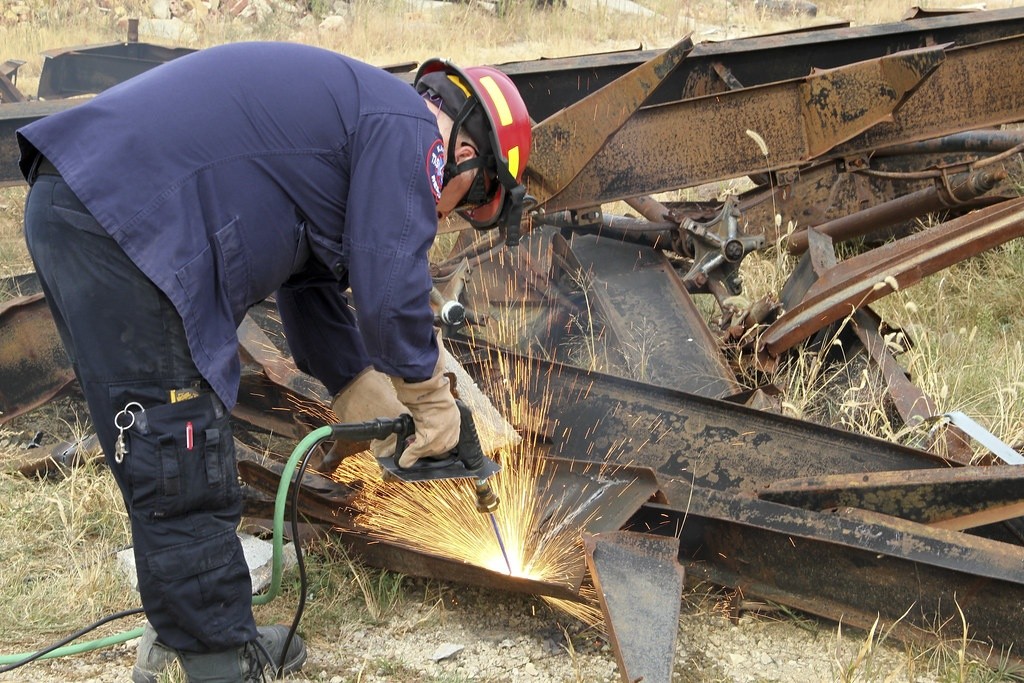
left=16, top=41, right=533, bottom=681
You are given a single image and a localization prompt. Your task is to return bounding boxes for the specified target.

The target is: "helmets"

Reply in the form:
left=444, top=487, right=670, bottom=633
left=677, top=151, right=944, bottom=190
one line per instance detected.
left=415, top=57, right=532, bottom=233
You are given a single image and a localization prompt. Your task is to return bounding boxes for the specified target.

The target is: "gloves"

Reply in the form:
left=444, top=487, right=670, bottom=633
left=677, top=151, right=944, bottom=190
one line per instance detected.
left=330, top=365, right=413, bottom=483
left=388, top=325, right=462, bottom=469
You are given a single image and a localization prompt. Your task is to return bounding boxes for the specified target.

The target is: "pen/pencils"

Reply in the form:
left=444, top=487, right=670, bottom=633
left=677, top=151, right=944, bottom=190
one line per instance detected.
left=185, top=421, right=194, bottom=451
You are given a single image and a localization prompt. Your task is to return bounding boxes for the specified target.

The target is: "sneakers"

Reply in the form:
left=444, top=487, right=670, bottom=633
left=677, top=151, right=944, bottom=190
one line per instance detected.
left=178, top=621, right=307, bottom=683
left=128, top=617, right=188, bottom=683
